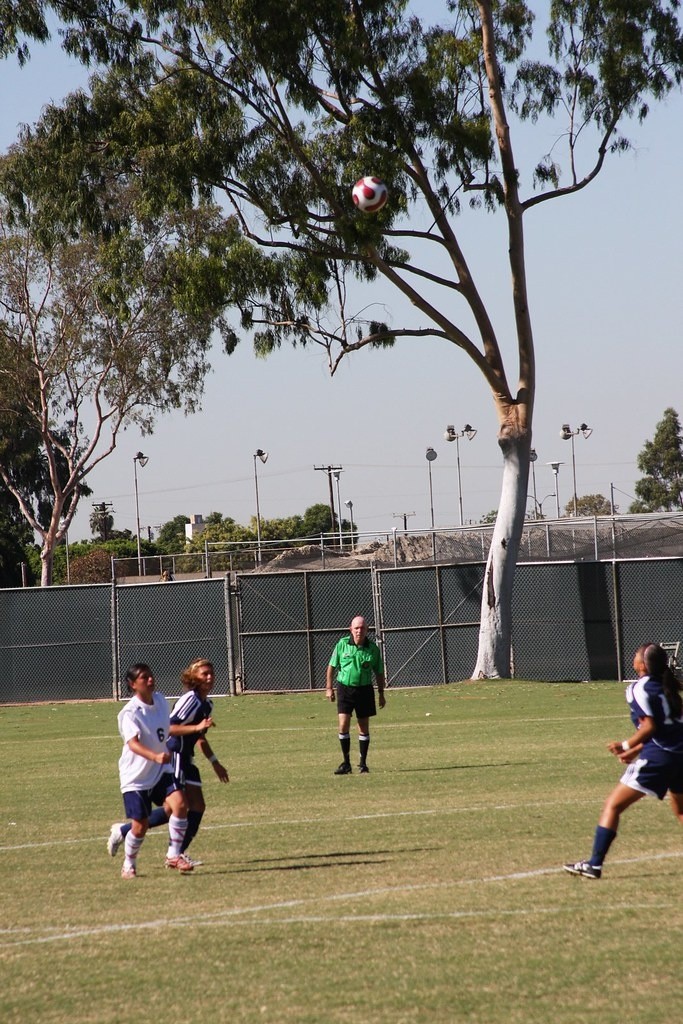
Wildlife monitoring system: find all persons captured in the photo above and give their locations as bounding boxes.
[326,616,386,774]
[108,659,229,867]
[117,664,194,878]
[560,646,683,880]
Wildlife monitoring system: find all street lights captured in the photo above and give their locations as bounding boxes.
[344,500,355,551]
[527,493,557,519]
[132,451,150,576]
[425,446,438,528]
[443,423,478,534]
[545,461,566,518]
[328,468,347,550]
[529,448,538,520]
[252,448,269,566]
[560,422,594,517]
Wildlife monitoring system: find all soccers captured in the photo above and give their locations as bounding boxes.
[352,176,388,214]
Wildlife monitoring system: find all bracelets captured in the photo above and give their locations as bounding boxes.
[622,741,629,751]
[209,755,216,762]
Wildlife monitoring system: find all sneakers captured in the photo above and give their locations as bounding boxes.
[106,823,125,857]
[563,860,602,879]
[335,763,352,774]
[164,854,194,870]
[181,853,202,865]
[120,865,136,879]
[357,765,369,773]
